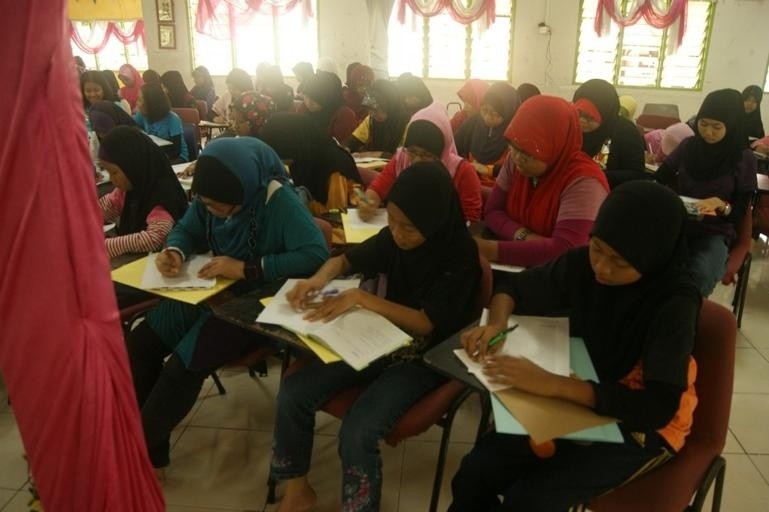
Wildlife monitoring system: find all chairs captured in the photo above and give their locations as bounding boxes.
[85,99,768,511]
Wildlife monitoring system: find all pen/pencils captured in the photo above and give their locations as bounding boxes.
[302,289,338,302]
[472,324,518,356]
[160,233,177,277]
[353,187,378,216]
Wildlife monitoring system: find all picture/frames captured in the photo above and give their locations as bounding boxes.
[154,1,174,22]
[157,22,178,49]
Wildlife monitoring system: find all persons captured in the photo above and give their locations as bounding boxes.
[269,158,487,511]
[125,136,329,490]
[445,179,705,511]
[73,57,768,309]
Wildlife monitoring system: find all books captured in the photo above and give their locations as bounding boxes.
[254,285,416,372]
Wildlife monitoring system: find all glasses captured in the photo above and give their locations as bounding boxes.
[402,147,437,161]
[576,109,596,122]
[189,192,235,215]
